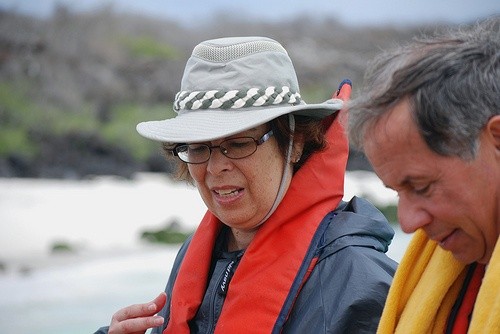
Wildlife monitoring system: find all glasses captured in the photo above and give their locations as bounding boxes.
[172,130,275,163]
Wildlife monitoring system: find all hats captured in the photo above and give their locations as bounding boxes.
[136,35,343,144]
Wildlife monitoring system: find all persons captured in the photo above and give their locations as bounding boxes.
[91,36,399,334]
[336,13,500,334]
